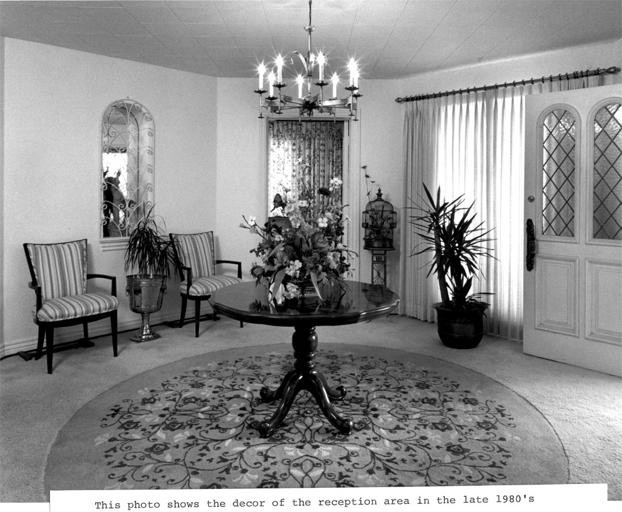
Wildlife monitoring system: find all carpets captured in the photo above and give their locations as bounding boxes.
[43,341,571,503]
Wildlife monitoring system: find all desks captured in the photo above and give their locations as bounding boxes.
[208,282,400,437]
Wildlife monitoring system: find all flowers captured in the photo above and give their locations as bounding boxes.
[239,177,359,314]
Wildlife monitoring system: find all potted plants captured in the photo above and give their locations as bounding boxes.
[123,203,170,342]
[401,181,501,348]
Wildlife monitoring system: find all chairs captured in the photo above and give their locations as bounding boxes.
[169,231,243,337]
[23,238,119,374]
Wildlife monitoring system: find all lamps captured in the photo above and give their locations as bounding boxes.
[254,0,364,121]
